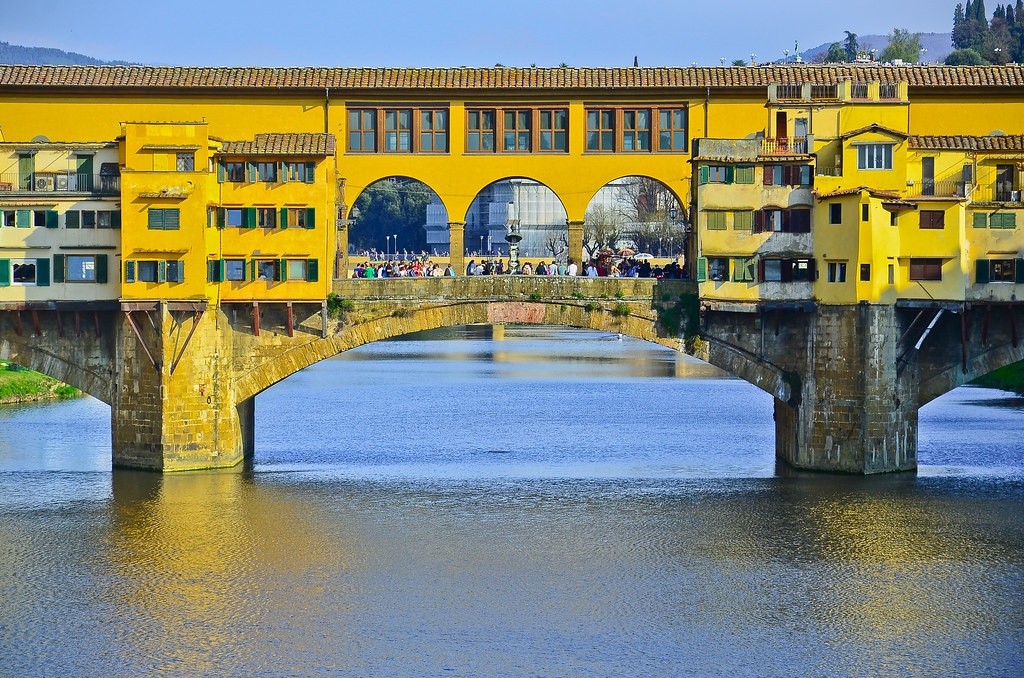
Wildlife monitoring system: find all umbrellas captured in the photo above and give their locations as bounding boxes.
[634,253,654,260]
[617,249,635,255]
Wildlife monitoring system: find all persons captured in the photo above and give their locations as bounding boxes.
[466,259,577,276]
[584,258,688,278]
[354,247,455,278]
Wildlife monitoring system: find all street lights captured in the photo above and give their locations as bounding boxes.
[670,237,673,257]
[720,56,727,65]
[659,237,662,257]
[994,48,1001,65]
[394,234,397,253]
[505,224,523,276]
[870,49,878,62]
[480,235,483,256]
[783,49,789,64]
[386,236,390,261]
[922,49,927,65]
